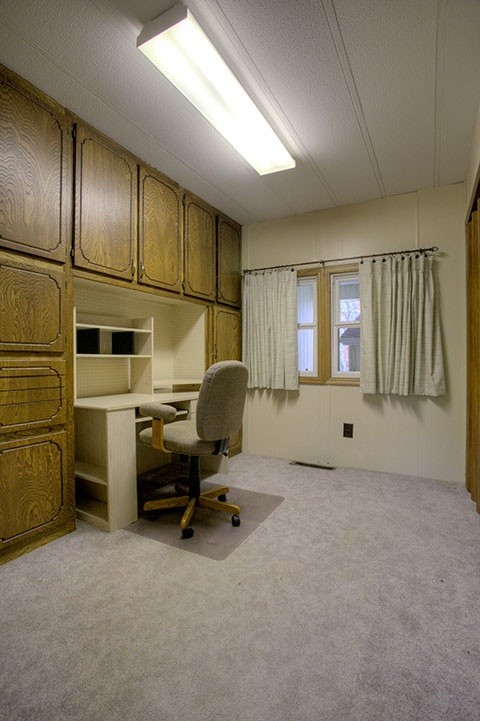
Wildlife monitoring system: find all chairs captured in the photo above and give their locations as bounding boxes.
[139,360,249,538]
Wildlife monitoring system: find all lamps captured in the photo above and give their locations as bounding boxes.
[135,5,296,177]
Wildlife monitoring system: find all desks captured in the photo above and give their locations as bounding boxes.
[73,391,228,532]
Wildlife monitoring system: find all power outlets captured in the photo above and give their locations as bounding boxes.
[343,423,353,438]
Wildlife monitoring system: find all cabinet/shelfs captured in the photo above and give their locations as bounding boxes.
[206,305,243,459]
[1,64,241,308]
[0,257,76,566]
[73,308,154,400]
